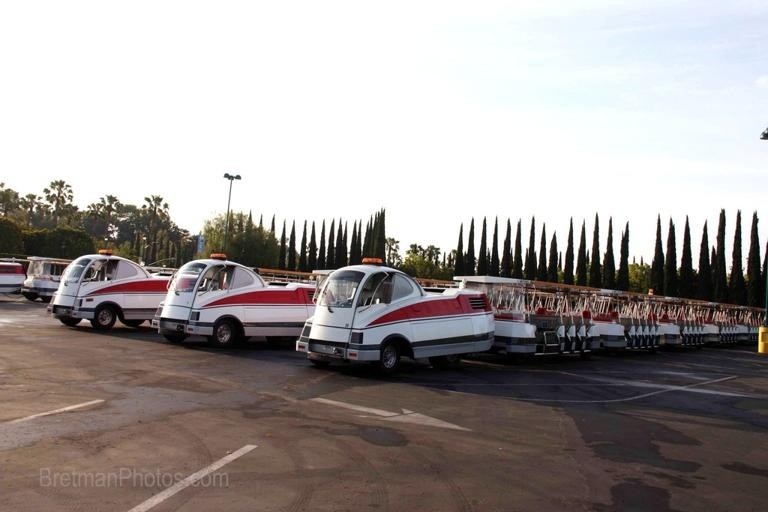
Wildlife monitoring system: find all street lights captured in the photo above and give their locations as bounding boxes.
[224,173,242,249]
[142,235,147,259]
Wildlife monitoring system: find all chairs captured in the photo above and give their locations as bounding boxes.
[361,283,385,305]
